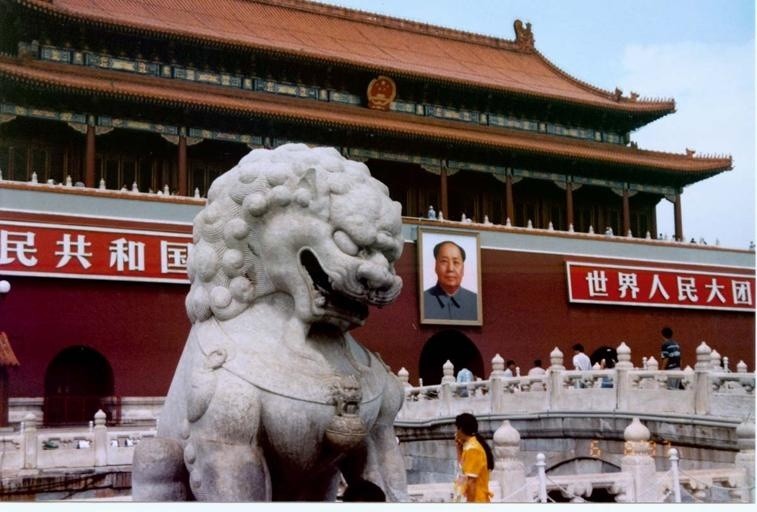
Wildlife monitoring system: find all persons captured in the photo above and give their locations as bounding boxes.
[525,359,547,392]
[454,362,475,395]
[571,343,594,388]
[424,241,478,320]
[454,412,492,503]
[660,326,684,388]
[600,353,617,388]
[502,359,517,392]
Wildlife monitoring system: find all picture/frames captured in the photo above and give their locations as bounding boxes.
[416,225,484,328]
